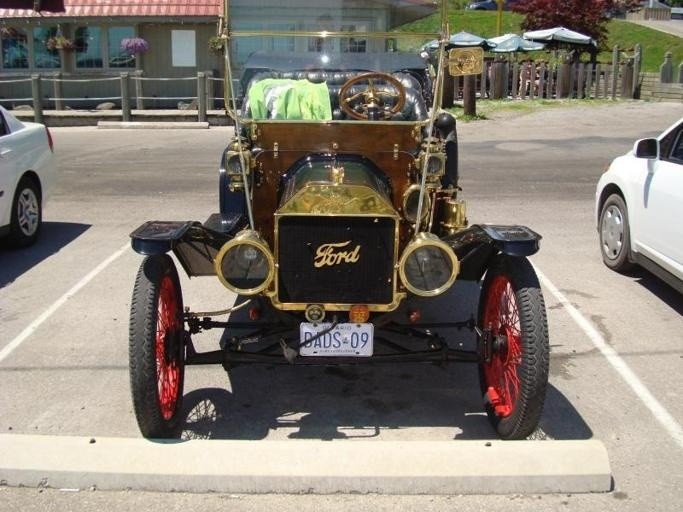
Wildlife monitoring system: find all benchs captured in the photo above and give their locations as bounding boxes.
[236,71,429,137]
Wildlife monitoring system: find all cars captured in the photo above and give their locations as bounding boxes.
[0,104,55,249]
[3,48,128,71]
[592,117,683,298]
[126,12,553,443]
[469,0,514,12]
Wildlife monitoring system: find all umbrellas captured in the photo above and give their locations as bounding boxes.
[421,39,439,51]
[487,33,544,61]
[522,25,599,67]
[437,28,495,50]
